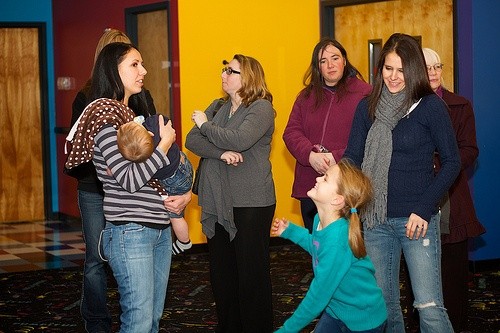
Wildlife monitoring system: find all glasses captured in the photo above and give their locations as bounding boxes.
[426,63,444,73]
[222,67,241,75]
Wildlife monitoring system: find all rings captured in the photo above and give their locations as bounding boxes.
[418,226,423,228]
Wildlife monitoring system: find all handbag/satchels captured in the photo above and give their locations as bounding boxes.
[192,98,225,195]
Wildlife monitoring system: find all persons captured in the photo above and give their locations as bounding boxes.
[407,47,483,333]
[282,39,375,233]
[63,29,156,333]
[272,158,388,333]
[185,54,277,333]
[90,42,193,333]
[117,114,193,256]
[336,32,459,333]
